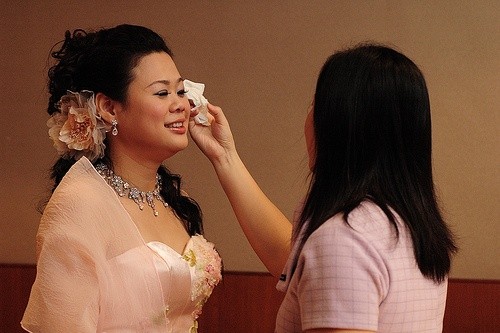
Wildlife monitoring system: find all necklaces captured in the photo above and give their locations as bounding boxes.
[95,162,168,216]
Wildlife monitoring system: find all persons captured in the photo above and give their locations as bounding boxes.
[19,23,225,333]
[187,37,463,333]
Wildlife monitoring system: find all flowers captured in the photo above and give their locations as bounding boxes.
[46,89,106,163]
